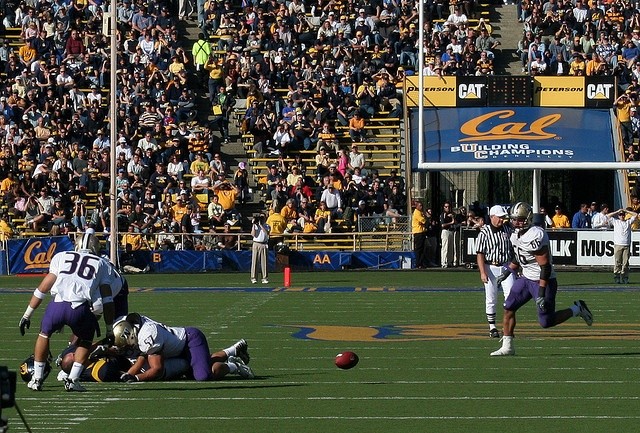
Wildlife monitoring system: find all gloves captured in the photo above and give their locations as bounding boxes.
[18,313,31,336]
[119,370,139,383]
[536,297,546,311]
[105,325,115,348]
[496,273,507,289]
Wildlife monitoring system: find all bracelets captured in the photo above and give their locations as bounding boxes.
[165,187,170,190]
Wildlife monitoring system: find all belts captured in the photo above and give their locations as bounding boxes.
[253,241,267,244]
[484,261,503,266]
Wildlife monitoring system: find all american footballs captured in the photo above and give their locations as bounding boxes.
[335,351,359,369]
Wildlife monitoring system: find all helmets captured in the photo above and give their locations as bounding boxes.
[509,202,534,230]
[74,233,102,256]
[20,350,52,384]
[108,320,138,356]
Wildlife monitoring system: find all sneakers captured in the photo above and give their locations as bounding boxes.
[574,299,594,326]
[489,328,499,338]
[63,378,87,392]
[251,279,258,284]
[489,347,515,356]
[623,278,630,283]
[228,356,255,379]
[233,338,250,365]
[27,378,44,391]
[262,280,269,284]
[614,278,620,283]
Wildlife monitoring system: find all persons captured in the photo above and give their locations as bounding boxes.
[628,105,640,133]
[136,101,160,128]
[266,165,282,192]
[50,105,65,128]
[54,128,72,151]
[1,13,15,28]
[88,36,109,57]
[292,155,306,180]
[91,191,111,232]
[190,170,209,193]
[201,0,220,36]
[35,61,49,84]
[43,87,56,105]
[108,313,250,382]
[99,57,111,85]
[588,201,600,216]
[18,135,36,151]
[20,171,34,195]
[250,213,271,284]
[569,52,586,76]
[596,31,609,45]
[490,202,593,356]
[189,151,209,176]
[39,187,54,215]
[96,151,111,177]
[516,1,573,34]
[175,122,191,138]
[25,90,39,107]
[137,131,159,154]
[57,159,74,182]
[536,29,545,43]
[520,31,534,72]
[622,40,640,68]
[79,53,99,80]
[455,206,468,226]
[171,196,191,222]
[630,194,640,209]
[116,136,134,158]
[289,111,312,150]
[280,198,298,221]
[11,76,25,97]
[19,38,37,66]
[118,1,133,26]
[47,55,60,69]
[266,206,288,236]
[133,204,147,223]
[623,144,640,162]
[538,207,552,228]
[549,54,569,76]
[528,34,547,60]
[591,204,613,231]
[571,204,591,228]
[579,32,596,54]
[597,36,619,67]
[35,117,52,140]
[142,190,159,218]
[69,84,86,110]
[336,148,350,176]
[220,1,500,114]
[4,84,12,97]
[141,33,154,55]
[612,94,635,152]
[529,54,549,74]
[1,170,21,195]
[8,89,22,104]
[20,7,40,33]
[558,29,576,51]
[82,158,100,179]
[269,182,289,211]
[32,30,54,55]
[72,149,88,175]
[73,257,129,346]
[132,6,153,38]
[20,233,115,391]
[426,208,439,268]
[118,130,137,144]
[127,154,144,184]
[60,352,255,383]
[212,180,239,213]
[169,43,190,63]
[156,32,169,49]
[0,115,9,133]
[53,151,73,171]
[467,211,484,228]
[170,31,182,48]
[17,149,36,171]
[24,104,43,121]
[349,111,365,143]
[188,130,208,153]
[68,113,85,142]
[66,182,85,200]
[24,188,44,230]
[159,126,175,140]
[40,145,58,161]
[620,0,639,39]
[15,1,27,25]
[606,209,638,283]
[61,54,80,76]
[54,5,70,33]
[19,114,34,131]
[165,137,187,158]
[118,54,200,129]
[626,76,640,93]
[43,16,56,38]
[5,126,19,143]
[11,98,27,120]
[192,33,212,92]
[46,171,61,192]
[343,167,407,223]
[476,205,519,337]
[5,136,19,154]
[210,154,228,179]
[157,224,175,248]
[156,5,176,37]
[6,61,20,82]
[412,202,428,268]
[86,85,103,107]
[211,85,237,145]
[586,53,602,76]
[0,96,13,118]
[348,145,366,174]
[550,205,571,228]
[124,31,140,54]
[208,195,225,222]
[71,0,112,30]
[167,154,184,182]
[121,225,142,251]
[0,212,13,243]
[2,147,16,164]
[605,2,625,29]
[291,177,313,201]
[56,69,74,85]
[143,149,162,162]
[52,198,66,222]
[315,147,331,182]
[320,184,344,215]
[92,129,110,151]
[624,206,640,230]
[440,202,455,269]
[150,162,173,194]
[66,30,84,55]
[317,122,341,153]
[274,154,289,179]
[549,36,569,64]
[272,125,289,151]
[88,109,103,131]
[182,205,193,243]
[0,39,15,64]
[315,202,331,234]
[631,61,640,84]
[286,165,302,187]
[69,200,87,229]
[588,1,604,28]
[573,0,588,24]
[297,198,312,227]
[5,182,26,214]
[234,162,249,206]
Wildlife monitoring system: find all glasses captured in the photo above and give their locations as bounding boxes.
[331,169,335,170]
[555,209,560,210]
[592,204,596,206]
[29,95,33,96]
[258,13,263,14]
[253,103,258,105]
[445,206,450,208]
[619,214,624,216]
[541,211,545,212]
[61,131,65,133]
[104,154,108,155]
[324,126,329,127]
[46,147,51,148]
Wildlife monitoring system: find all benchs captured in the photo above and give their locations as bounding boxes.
[235,111,401,177]
[6,28,26,54]
[78,89,111,106]
[1,193,247,252]
[284,220,410,250]
[624,142,640,190]
[432,3,493,35]
[273,89,289,100]
[210,35,226,54]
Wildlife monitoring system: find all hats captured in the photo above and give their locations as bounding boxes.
[340,77,346,81]
[217,173,225,175]
[180,190,187,195]
[0,96,6,101]
[193,125,202,133]
[327,164,338,169]
[185,204,194,208]
[359,200,366,205]
[96,129,105,135]
[124,189,130,194]
[555,205,561,208]
[8,136,13,140]
[22,114,28,120]
[29,188,38,193]
[60,68,66,72]
[291,165,298,170]
[118,137,126,143]
[343,56,349,60]
[13,89,18,93]
[352,144,358,148]
[340,16,346,20]
[40,61,46,65]
[250,31,256,34]
[358,18,365,21]
[359,8,366,13]
[37,13,43,18]
[9,125,16,128]
[328,184,334,187]
[446,45,453,50]
[617,210,625,214]
[179,122,186,126]
[22,150,28,155]
[297,111,303,116]
[490,205,508,217]
[353,110,359,114]
[356,31,362,36]
[45,144,51,147]
[4,39,9,43]
[338,30,344,33]
[433,65,441,72]
[173,137,180,142]
[171,30,178,34]
[302,198,308,202]
[143,102,152,106]
[380,68,387,73]
[398,66,404,71]
[278,47,284,52]
[316,80,322,86]
[298,83,303,87]
[409,24,416,28]
[91,84,97,88]
[276,17,283,21]
[320,146,325,150]
[118,169,126,172]
[271,165,276,168]
[306,47,318,54]
[337,149,346,154]
[9,54,15,57]
[161,6,168,12]
[239,162,246,169]
[228,55,237,60]
[15,76,22,80]
[176,196,182,200]
[55,197,61,202]
[329,12,335,16]
[287,98,292,103]
